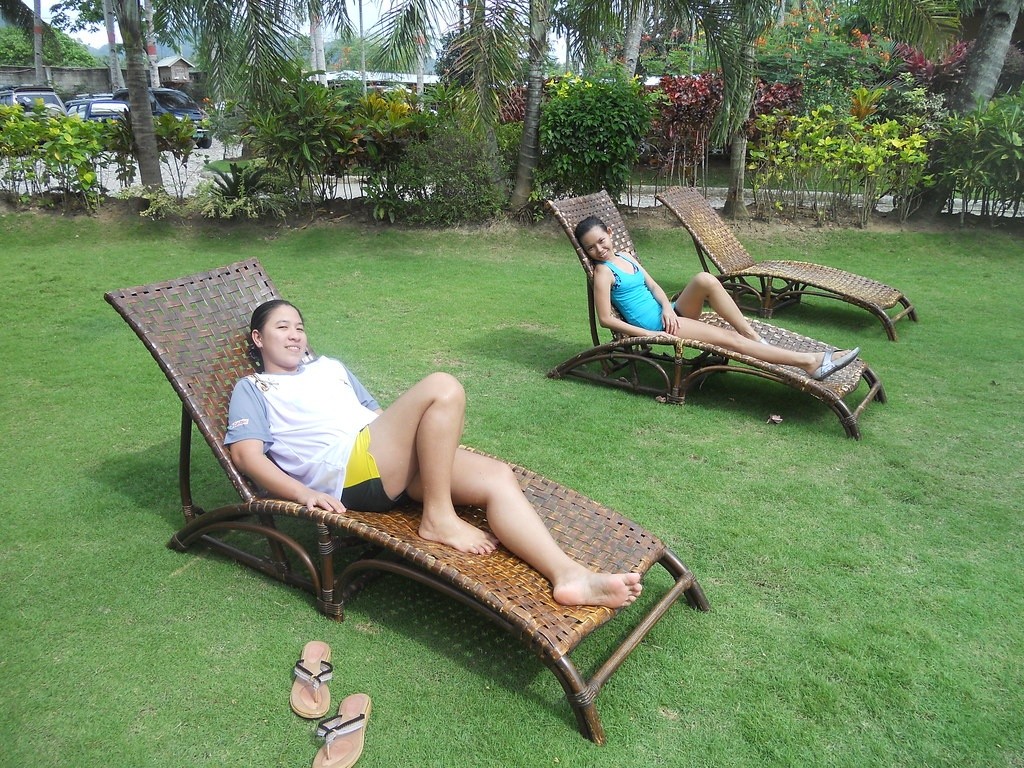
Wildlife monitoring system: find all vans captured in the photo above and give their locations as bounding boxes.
[112,87,213,151]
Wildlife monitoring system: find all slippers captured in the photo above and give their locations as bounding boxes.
[312,693,371,768]
[814,347,860,380]
[290,641,333,719]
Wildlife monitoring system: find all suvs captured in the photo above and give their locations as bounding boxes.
[0,83,69,121]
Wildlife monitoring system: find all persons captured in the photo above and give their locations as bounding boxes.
[576,215,860,381]
[225,300,642,610]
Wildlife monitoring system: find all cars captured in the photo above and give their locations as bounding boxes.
[65,100,129,124]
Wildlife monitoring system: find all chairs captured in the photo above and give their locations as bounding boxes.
[654,185,921,343]
[104,255,711,746]
[544,190,887,442]
